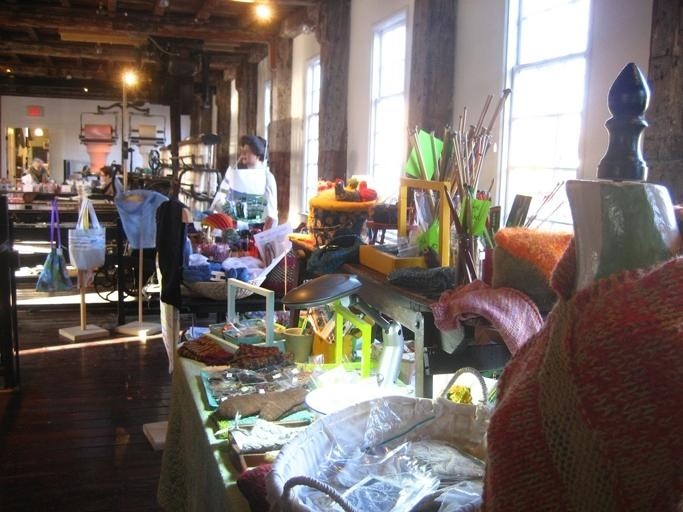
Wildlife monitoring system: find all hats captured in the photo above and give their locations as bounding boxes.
[238,134,267,162]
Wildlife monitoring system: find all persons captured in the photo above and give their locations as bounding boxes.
[28,157,50,185]
[209,134,278,231]
[99,165,117,199]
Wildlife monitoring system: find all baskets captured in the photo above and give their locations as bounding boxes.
[182,274,266,299]
[272,393,494,512]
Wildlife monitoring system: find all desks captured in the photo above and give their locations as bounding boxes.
[175,295,290,325]
[286,231,491,402]
[155,341,497,512]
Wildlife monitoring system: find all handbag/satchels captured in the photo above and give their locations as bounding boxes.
[35,254,72,293]
[68,229,105,270]
